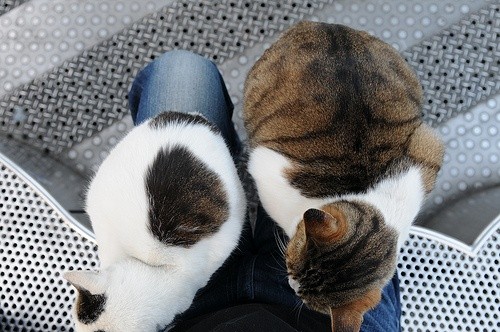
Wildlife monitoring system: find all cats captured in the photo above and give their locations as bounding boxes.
[62,112,246,332]
[245,21,445,332]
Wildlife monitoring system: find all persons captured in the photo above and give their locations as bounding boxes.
[128,50,401,332]
